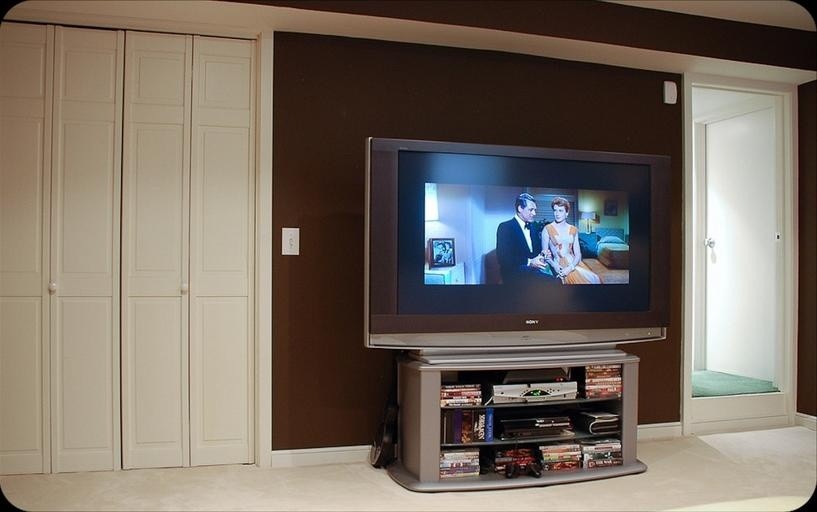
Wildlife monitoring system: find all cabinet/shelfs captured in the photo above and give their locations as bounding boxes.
[0,22,256,477]
[385,353,648,494]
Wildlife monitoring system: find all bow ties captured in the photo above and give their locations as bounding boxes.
[525,221,531,230]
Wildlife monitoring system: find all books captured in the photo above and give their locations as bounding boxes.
[437,362,623,478]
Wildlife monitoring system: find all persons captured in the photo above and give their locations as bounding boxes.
[541,196,603,286]
[433,242,446,263]
[495,192,561,286]
[441,241,454,265]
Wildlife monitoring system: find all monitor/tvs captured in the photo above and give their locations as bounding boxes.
[364,136,671,365]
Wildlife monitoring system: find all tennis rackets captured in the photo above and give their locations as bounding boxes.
[370,403,397,467]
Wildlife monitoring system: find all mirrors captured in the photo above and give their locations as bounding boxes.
[679,71,800,436]
